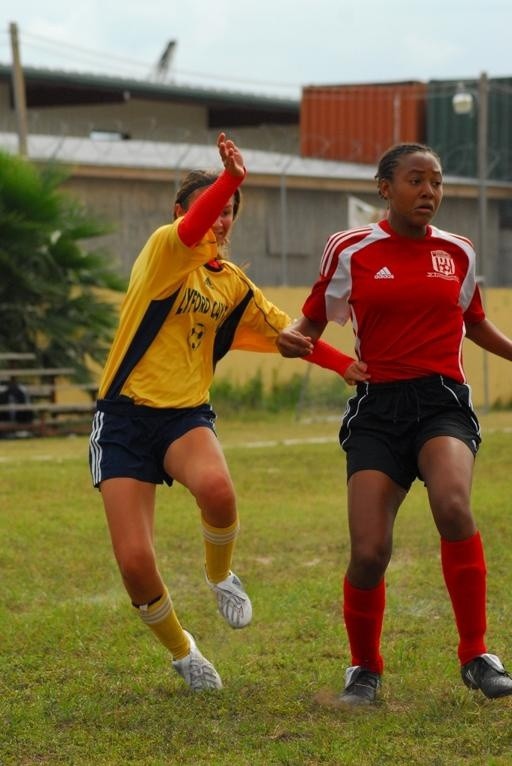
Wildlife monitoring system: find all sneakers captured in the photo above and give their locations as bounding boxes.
[204,559,253,629]
[340,665,383,709]
[458,652,512,699]
[171,629,222,697]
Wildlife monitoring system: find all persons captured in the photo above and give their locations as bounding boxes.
[87,132,374,694]
[278,141,510,702]
